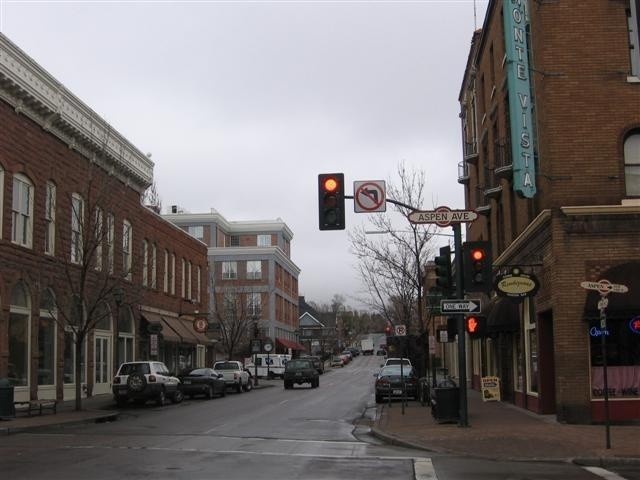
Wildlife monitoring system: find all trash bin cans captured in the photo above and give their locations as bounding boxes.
[0,378,16,418]
[431,387,461,423]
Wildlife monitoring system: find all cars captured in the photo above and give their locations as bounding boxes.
[181,367,228,399]
[330,346,361,368]
[212,360,253,393]
[373,358,421,404]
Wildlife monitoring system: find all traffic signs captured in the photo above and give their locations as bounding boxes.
[438,299,482,315]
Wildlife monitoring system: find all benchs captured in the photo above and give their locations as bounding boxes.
[13,398,58,419]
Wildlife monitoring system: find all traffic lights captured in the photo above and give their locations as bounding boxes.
[434,245,454,296]
[462,240,493,292]
[467,317,486,340]
[317,172,346,231]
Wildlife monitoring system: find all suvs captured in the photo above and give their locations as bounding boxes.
[283,359,320,390]
[111,359,184,405]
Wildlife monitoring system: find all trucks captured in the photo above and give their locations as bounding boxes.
[243,353,293,380]
